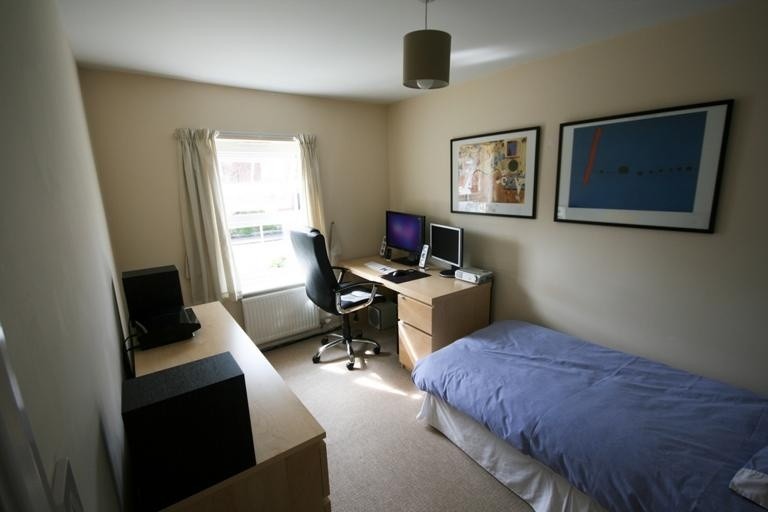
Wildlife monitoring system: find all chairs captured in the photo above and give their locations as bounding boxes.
[289,224,386,371]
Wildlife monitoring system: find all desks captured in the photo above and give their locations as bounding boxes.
[340,256,494,370]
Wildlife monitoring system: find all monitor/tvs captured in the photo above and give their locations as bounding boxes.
[429,221,464,278]
[386,210,425,266]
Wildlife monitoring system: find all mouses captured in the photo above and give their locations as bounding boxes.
[392,269,406,277]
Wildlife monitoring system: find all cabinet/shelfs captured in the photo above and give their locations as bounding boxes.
[128,300,333,512]
[396,282,492,370]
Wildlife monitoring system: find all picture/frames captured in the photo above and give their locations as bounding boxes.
[450,99,735,234]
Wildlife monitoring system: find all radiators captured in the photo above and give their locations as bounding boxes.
[240,285,331,346]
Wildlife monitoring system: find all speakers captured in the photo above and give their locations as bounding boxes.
[379,235,388,256]
[418,244,430,268]
[120,350,257,512]
[367,302,397,330]
[121,264,184,329]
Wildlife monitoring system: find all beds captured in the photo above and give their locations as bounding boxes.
[412,319,768,512]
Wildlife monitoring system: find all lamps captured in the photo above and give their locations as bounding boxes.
[403,1,451,91]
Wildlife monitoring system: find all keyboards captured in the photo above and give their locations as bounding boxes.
[364,260,397,275]
